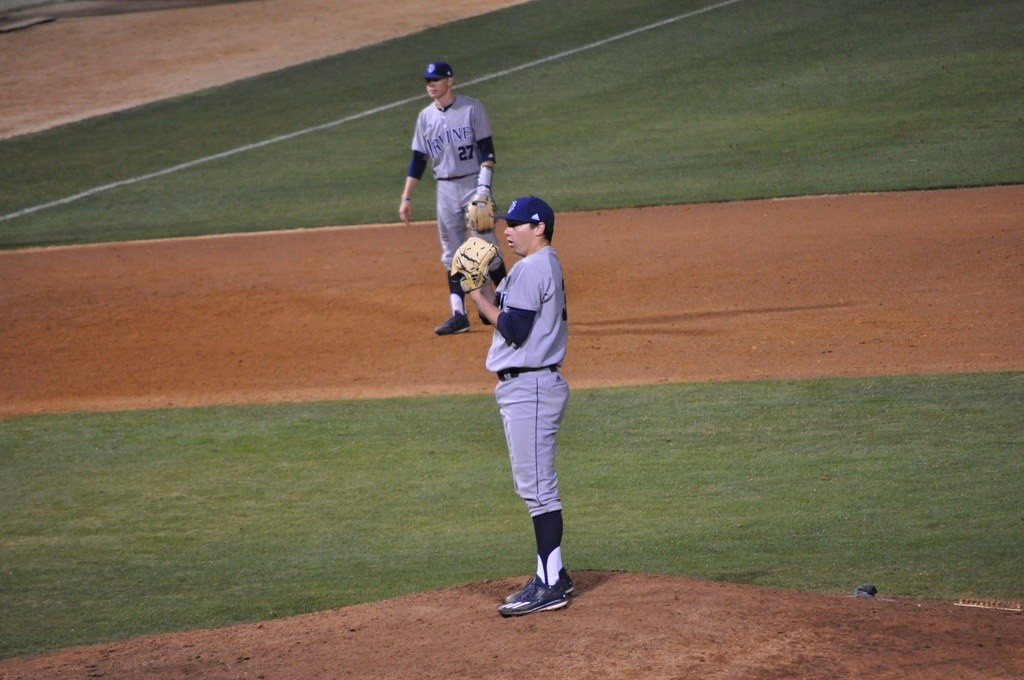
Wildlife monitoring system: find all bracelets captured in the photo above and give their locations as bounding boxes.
[401,198,410,201]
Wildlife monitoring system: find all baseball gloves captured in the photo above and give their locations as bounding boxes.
[449,235,500,294]
[465,191,499,235]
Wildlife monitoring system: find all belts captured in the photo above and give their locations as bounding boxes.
[498,365,556,381]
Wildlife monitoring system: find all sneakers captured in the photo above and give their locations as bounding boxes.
[434,311,470,334]
[499,573,567,614]
[503,567,575,603]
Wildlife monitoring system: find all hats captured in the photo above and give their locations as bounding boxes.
[491,196,555,227]
[421,61,453,79]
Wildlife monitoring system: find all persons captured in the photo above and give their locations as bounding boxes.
[399,61,507,335]
[451,196,575,617]
